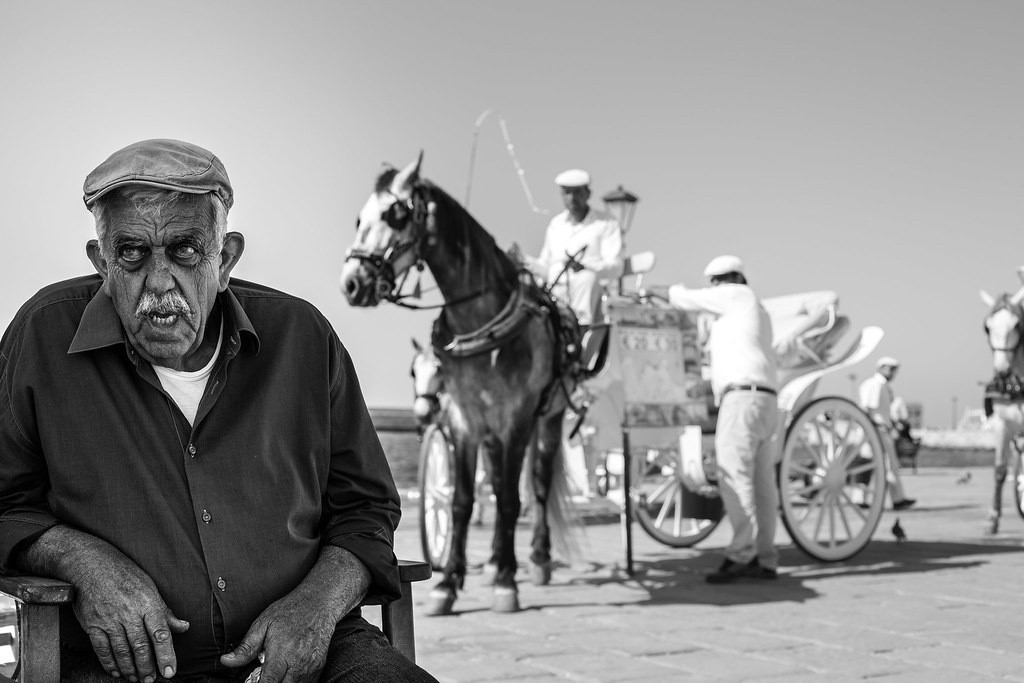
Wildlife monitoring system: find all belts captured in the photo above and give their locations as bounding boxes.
[725,385,777,394]
[992,349,1015,351]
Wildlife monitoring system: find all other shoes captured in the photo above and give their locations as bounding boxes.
[706,558,743,584]
[750,567,777,581]
[894,499,915,509]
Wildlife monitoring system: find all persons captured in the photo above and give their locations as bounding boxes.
[0,139,439,683]
[633,256,782,584]
[857,356,918,511]
[509,169,625,380]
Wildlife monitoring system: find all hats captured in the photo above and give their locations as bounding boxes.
[878,356,899,367]
[82,138,234,215]
[704,255,743,276]
[554,169,590,188]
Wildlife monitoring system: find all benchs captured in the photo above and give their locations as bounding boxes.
[763,287,841,367]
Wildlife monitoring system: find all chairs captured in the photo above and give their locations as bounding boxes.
[605,251,655,307]
[0,558,434,683]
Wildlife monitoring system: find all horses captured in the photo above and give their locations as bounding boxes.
[338,148,583,617]
[976,283,1023,536]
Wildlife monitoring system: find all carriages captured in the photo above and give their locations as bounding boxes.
[335,148,896,625]
[406,335,633,574]
[976,263,1024,538]
